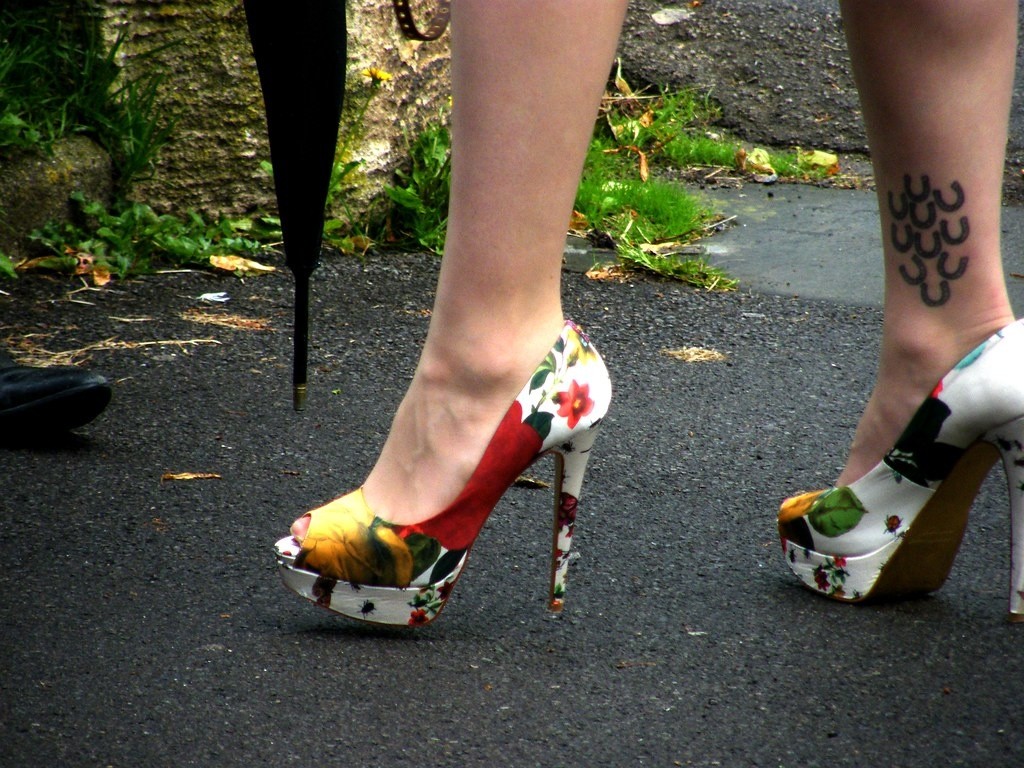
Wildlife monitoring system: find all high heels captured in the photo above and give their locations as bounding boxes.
[778,318,1024,622]
[272,317,613,625]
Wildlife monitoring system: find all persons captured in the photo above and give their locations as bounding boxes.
[0,346,112,435]
[272,0,1023,621]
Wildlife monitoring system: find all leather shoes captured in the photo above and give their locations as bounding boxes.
[0,347,112,438]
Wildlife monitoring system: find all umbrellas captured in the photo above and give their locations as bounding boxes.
[243,0,347,411]
